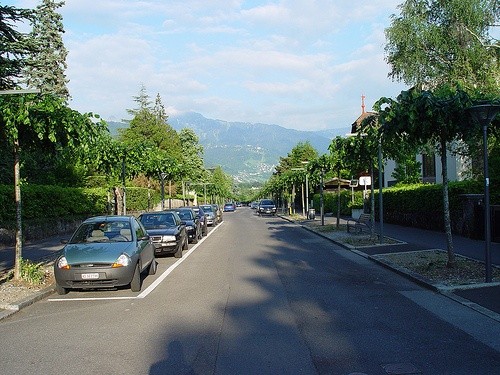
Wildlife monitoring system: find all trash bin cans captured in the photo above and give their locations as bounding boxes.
[309,209,315,220]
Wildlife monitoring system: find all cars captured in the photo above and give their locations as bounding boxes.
[54,215,157,295]
[248,203,252,207]
[179,206,208,236]
[199,204,218,227]
[163,208,202,244]
[224,203,235,212]
[236,204,241,207]
[210,204,223,223]
[136,211,189,258]
[251,202,258,209]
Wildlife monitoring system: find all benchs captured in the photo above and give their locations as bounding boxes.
[347,213,373,235]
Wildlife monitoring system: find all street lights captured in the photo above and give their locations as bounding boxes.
[465,103,500,284]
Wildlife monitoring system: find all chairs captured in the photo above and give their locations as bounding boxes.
[86,230,109,243]
[116,229,133,242]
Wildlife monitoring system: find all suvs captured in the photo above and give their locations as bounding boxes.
[256,199,277,216]
